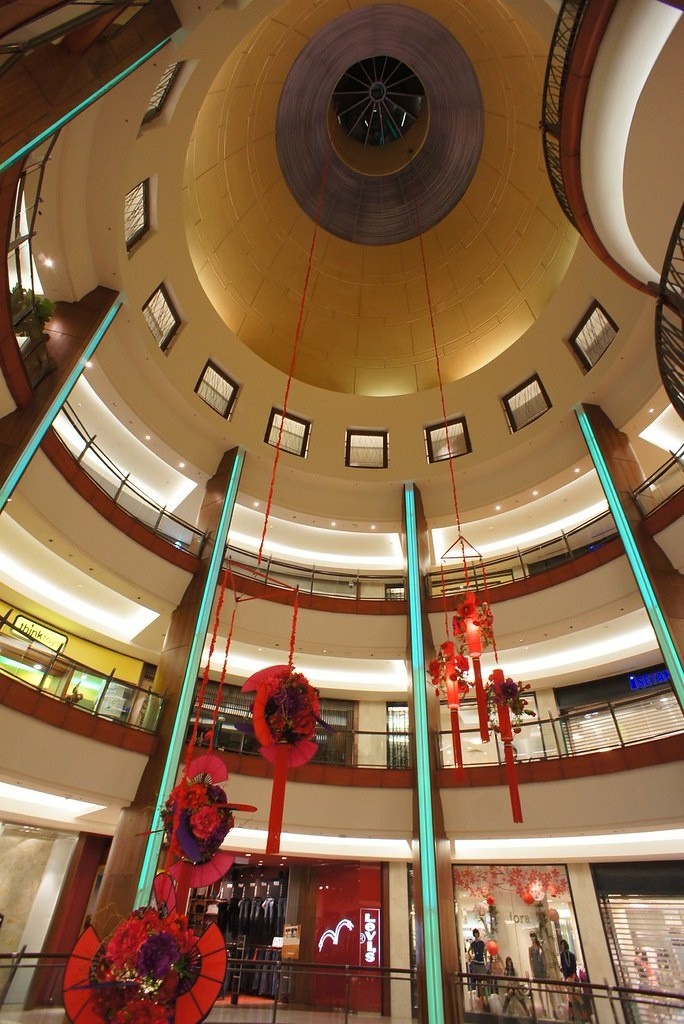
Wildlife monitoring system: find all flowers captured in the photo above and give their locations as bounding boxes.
[92,905,200,1024]
[458,599,478,619]
[160,777,235,864]
[472,601,494,648]
[458,679,478,703]
[484,674,536,734]
[234,677,337,744]
[432,676,446,697]
[426,656,446,676]
[453,643,470,676]
[449,673,458,681]
[452,616,467,637]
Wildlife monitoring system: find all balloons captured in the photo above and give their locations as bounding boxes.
[522,893,533,905]
[549,907,558,922]
[487,941,498,957]
[487,896,494,907]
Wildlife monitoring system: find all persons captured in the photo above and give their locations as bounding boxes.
[559,939,578,1022]
[467,928,488,998]
[501,956,531,1018]
[528,931,559,1020]
